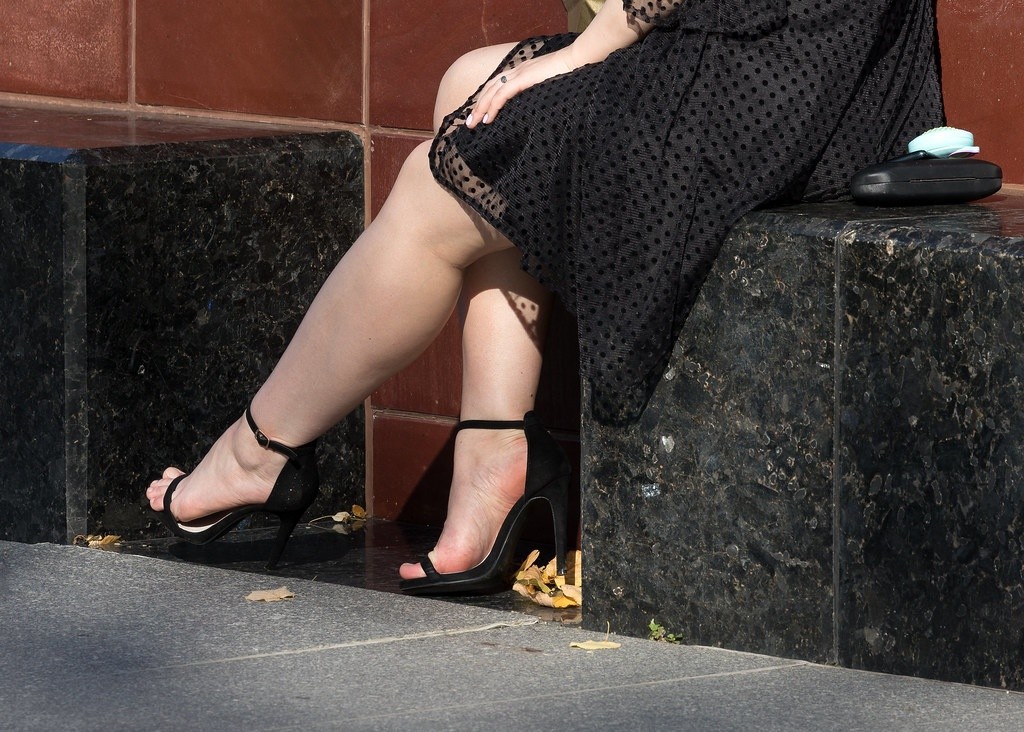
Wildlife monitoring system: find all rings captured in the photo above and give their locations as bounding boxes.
[501,76,507,83]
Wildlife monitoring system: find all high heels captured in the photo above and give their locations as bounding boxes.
[395,413,567,600]
[161,404,319,558]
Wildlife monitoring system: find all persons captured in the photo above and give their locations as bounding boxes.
[146,0,947,592]
